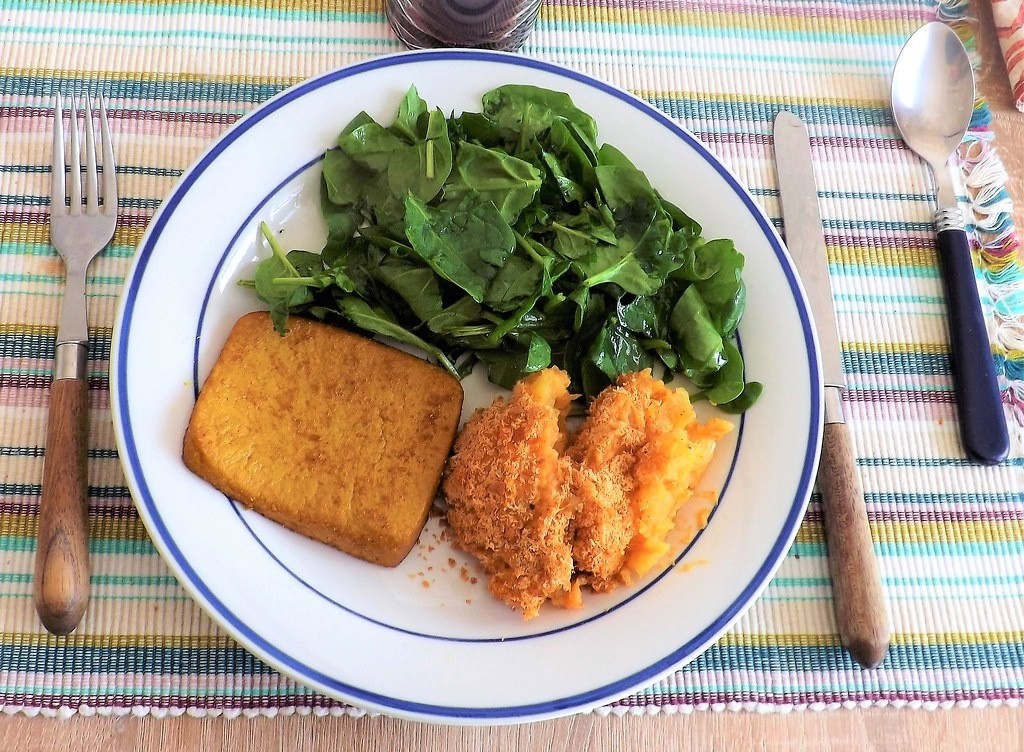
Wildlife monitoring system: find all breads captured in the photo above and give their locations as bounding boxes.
[182,312,463,567]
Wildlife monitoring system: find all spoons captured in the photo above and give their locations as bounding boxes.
[890,22,1010,466]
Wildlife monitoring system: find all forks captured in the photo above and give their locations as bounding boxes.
[36,93,118,634]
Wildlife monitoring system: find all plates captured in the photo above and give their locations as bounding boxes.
[109,48,826,727]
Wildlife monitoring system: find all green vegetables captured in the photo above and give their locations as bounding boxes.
[239,83,763,415]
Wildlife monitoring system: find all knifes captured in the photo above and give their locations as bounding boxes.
[773,110,889,669]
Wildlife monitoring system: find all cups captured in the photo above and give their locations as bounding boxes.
[384,0,543,52]
